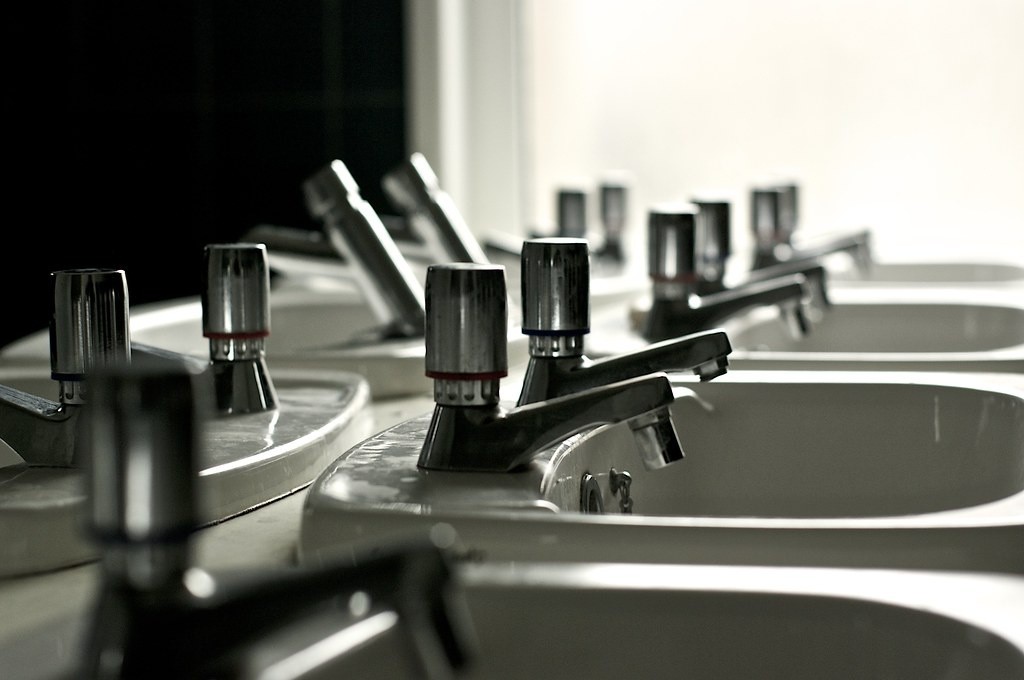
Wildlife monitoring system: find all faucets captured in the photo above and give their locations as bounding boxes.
[417,263,683,474]
[364,149,517,312]
[57,357,486,680]
[745,183,875,274]
[247,158,427,343]
[0,266,136,474]
[127,240,283,421]
[645,197,832,345]
[512,236,733,408]
[478,178,631,280]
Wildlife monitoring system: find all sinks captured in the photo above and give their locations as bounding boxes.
[538,381,1024,519]
[129,301,387,357]
[258,579,1024,680]
[729,303,1024,353]
[0,373,65,471]
[815,261,1024,288]
